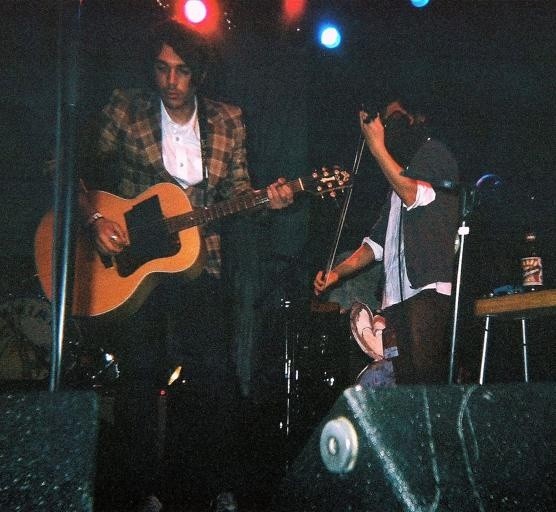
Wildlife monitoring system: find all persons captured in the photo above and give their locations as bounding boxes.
[312,92,461,386]
[43,18,294,511]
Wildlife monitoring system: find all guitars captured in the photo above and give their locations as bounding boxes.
[35,164,351,323]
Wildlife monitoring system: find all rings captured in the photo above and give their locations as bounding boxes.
[282,195,288,203]
[109,235,118,242]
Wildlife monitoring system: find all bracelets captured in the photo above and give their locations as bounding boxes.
[84,213,104,225]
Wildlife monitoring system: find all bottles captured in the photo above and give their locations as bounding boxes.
[521,232,544,293]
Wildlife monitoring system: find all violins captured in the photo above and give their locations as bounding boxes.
[360,111,425,171]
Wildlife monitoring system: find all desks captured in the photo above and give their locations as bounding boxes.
[473,288,556,385]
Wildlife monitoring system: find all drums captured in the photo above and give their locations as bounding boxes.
[1,290,90,387]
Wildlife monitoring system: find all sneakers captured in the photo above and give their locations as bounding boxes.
[209,491,240,512]
[134,494,163,512]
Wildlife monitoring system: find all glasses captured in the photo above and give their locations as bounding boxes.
[381,110,409,121]
